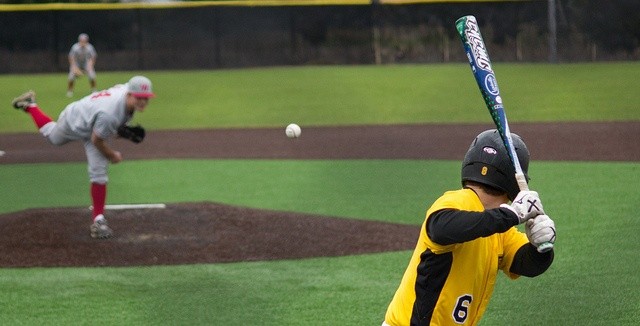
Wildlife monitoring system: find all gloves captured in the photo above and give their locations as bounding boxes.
[525,215,556,247]
[500,190,544,224]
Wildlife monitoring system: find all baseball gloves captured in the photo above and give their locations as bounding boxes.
[117,125,145,143]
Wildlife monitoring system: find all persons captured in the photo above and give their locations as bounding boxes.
[14,76,157,238]
[67,33,97,97]
[381,129,556,326]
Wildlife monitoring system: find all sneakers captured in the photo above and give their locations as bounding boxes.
[12,90,35,109]
[90,218,113,238]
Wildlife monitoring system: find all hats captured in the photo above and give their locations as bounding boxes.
[127,76,157,98]
[78,33,89,42]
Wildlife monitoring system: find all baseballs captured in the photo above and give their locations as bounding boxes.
[285,124,301,138]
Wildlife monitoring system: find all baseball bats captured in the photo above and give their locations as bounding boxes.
[454,15,553,254]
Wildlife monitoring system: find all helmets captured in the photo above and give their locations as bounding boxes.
[462,128,529,202]
